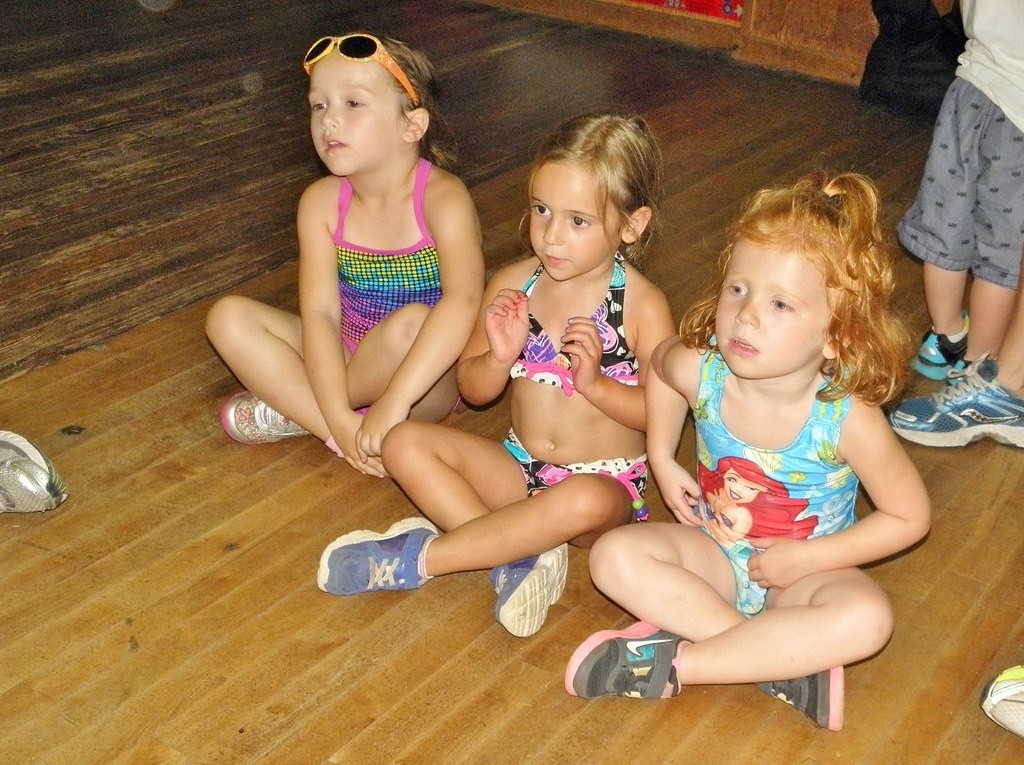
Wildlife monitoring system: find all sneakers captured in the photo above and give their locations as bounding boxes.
[489,543,568,637]
[888,350,1024,447]
[317,517,438,596]
[220,391,312,445]
[0,430,69,513]
[565,621,695,699]
[757,666,844,730]
[980,665,1024,739]
[911,311,973,386]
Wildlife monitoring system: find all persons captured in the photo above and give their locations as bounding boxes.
[880,0,1024,394]
[560,164,934,734]
[311,109,676,639]
[198,30,483,482]
[885,234,1024,455]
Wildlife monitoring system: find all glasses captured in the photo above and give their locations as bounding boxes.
[304,34,419,106]
